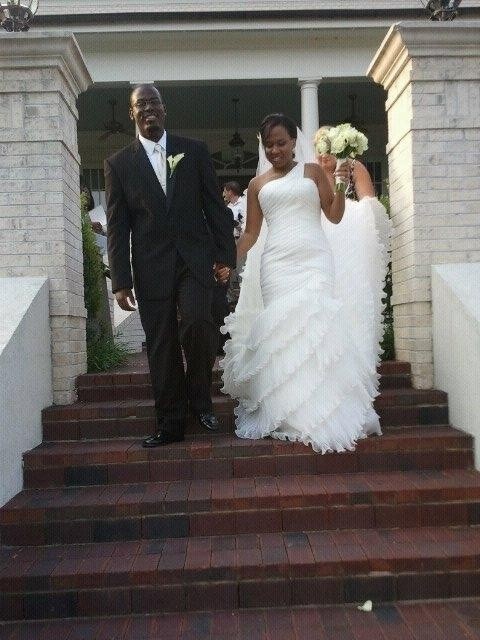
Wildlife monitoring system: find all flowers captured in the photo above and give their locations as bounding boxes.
[313,122,369,193]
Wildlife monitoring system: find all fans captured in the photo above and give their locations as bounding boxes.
[97,98,135,140]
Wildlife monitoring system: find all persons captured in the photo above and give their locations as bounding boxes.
[80,184,113,280]
[221,180,251,304]
[213,111,396,453]
[313,125,375,203]
[102,81,236,451]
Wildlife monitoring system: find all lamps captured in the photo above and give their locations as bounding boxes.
[0,0,39,32]
[228,97,245,172]
[421,0,462,21]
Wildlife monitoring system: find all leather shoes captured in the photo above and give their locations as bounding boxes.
[198,410,219,431]
[143,430,184,446]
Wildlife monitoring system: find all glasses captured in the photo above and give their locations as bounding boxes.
[132,99,162,108]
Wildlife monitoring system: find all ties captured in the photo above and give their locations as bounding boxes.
[154,145,167,196]
